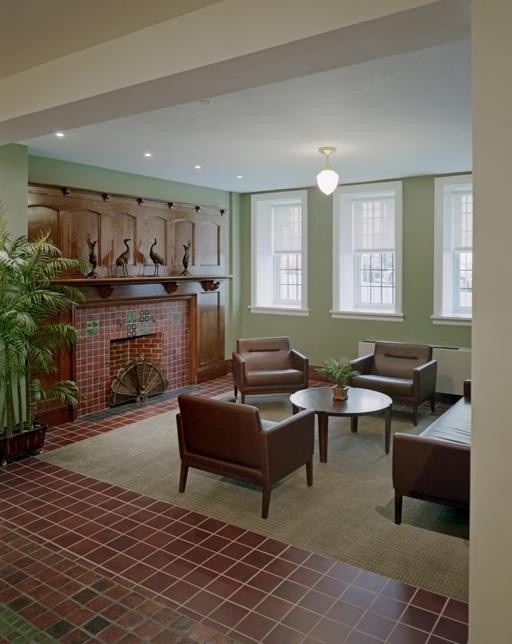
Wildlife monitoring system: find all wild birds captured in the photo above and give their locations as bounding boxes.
[148,237,168,277]
[116,238,133,278]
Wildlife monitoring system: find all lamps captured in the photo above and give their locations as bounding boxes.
[316,146,341,195]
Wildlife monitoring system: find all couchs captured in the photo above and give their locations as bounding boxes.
[394,380,469,523]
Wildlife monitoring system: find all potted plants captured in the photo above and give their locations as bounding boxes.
[0,205,83,464]
[317,357,358,401]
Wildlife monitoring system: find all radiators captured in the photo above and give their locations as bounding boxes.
[358,339,471,397]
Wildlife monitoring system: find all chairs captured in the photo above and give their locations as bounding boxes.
[231,337,310,403]
[174,394,317,519]
[347,343,440,426]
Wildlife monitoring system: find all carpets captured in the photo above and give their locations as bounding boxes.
[36,391,471,603]
